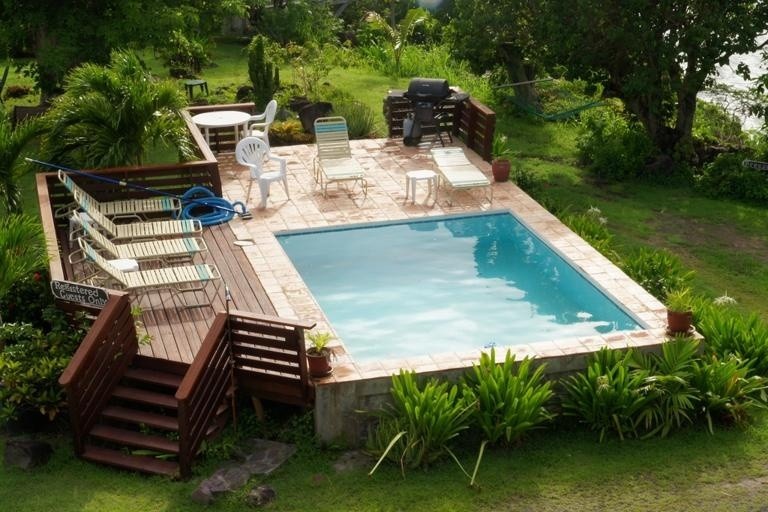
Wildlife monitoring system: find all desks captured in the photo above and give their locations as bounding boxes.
[186,80,208,99]
[406,170,439,202]
[193,111,252,146]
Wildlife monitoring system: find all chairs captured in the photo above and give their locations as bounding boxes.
[239,99,278,150]
[431,147,494,207]
[313,116,368,198]
[236,137,292,208]
[54,169,223,310]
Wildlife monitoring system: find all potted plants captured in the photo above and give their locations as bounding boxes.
[663,286,696,337]
[305,331,338,377]
[490,133,517,182]
